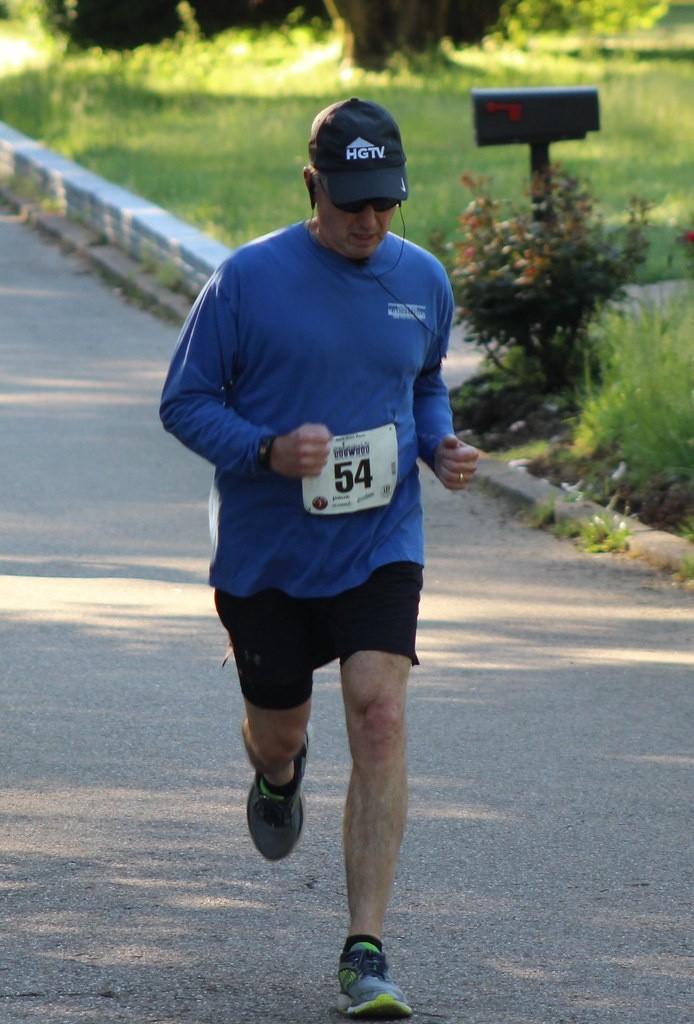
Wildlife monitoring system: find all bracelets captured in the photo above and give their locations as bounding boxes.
[258,434,275,469]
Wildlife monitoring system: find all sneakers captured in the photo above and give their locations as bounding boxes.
[327,949,411,1017]
[245,732,310,862]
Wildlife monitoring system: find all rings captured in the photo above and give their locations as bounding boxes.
[459,473,463,482]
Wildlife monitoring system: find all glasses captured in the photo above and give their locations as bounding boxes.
[315,172,403,214]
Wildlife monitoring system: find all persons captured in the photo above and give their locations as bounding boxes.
[160,97,479,1020]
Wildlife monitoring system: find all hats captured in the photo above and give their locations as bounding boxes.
[308,97,409,205]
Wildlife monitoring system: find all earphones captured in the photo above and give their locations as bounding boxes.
[308,175,316,210]
